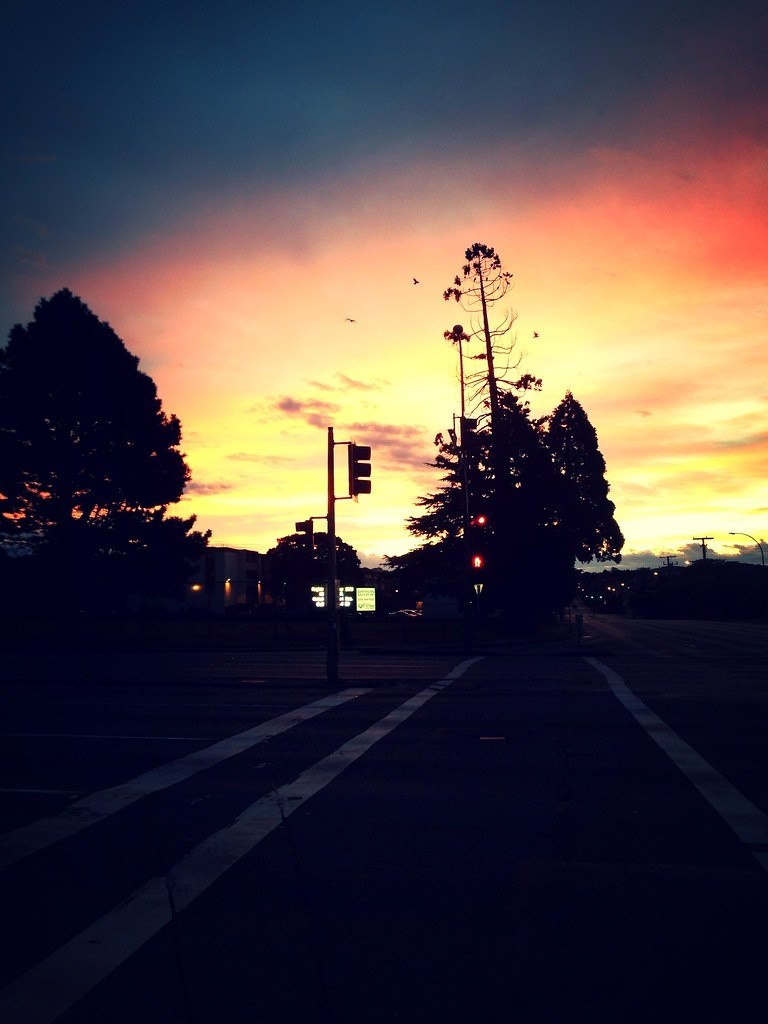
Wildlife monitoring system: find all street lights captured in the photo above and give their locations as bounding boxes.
[730,532,765,565]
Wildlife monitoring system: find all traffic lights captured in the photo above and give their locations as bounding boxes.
[296,521,314,562]
[471,552,485,571]
[348,443,372,496]
[475,515,489,527]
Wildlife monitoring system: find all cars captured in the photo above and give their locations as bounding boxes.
[388,608,423,619]
